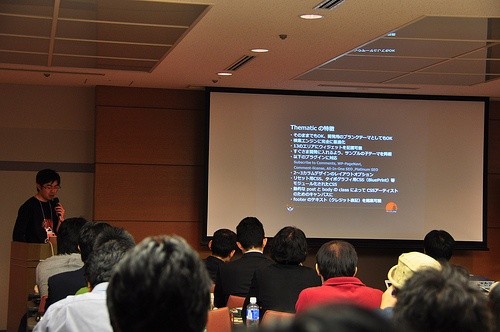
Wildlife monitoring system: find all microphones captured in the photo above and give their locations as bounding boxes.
[54,197,61,220]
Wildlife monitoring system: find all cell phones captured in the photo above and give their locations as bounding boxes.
[384,279,396,295]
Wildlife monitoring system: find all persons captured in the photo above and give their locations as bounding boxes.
[12,169,65,243]
[31,216,499,332]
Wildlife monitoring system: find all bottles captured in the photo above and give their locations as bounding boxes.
[246,297,259,332]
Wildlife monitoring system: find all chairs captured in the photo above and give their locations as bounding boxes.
[19,292,295,332]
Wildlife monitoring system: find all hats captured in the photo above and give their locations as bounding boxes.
[388,252,442,289]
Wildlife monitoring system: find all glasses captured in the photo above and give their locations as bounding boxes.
[43,185,61,189]
